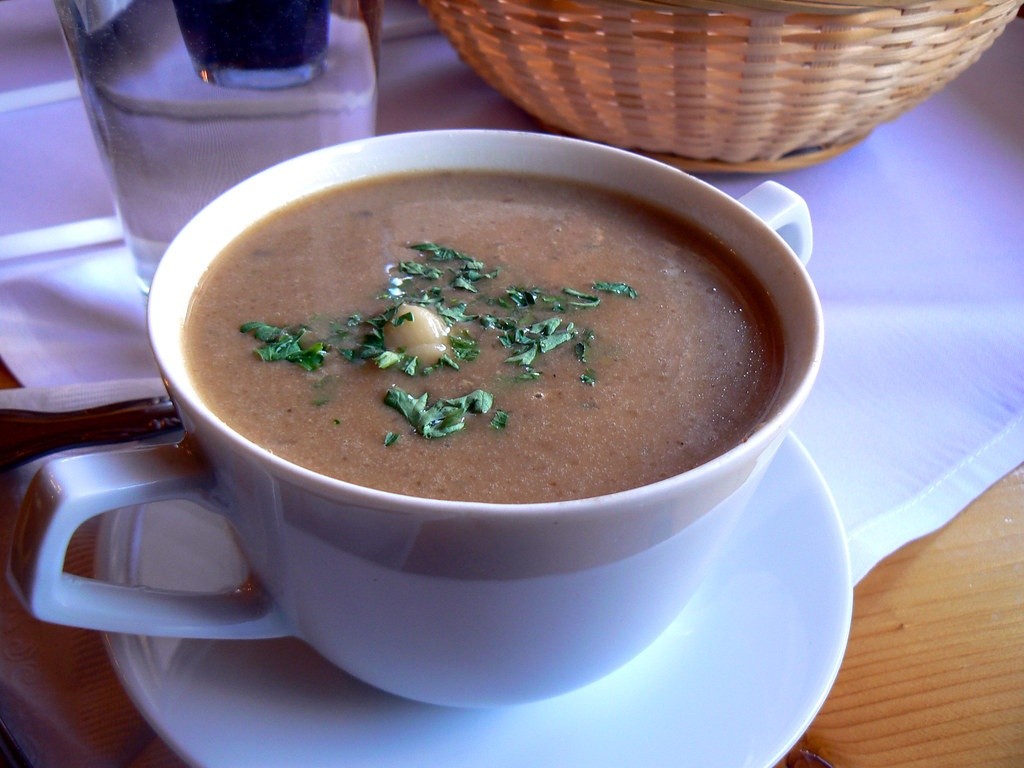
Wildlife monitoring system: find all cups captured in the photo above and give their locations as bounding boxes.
[55,0,379,301]
[6,126,829,714]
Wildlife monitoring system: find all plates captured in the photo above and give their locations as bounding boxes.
[90,427,857,768]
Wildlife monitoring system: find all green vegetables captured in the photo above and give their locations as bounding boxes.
[240,240,640,448]
